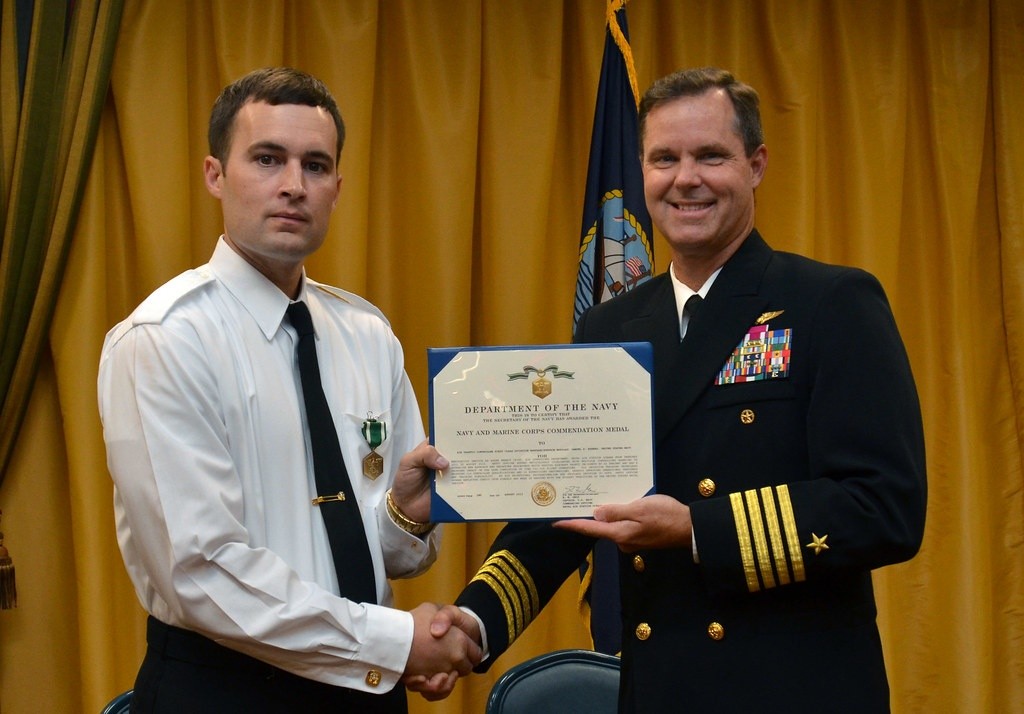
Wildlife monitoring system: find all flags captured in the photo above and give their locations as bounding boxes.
[571,0,654,656]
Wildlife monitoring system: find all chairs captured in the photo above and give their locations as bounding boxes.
[484,649,620,714]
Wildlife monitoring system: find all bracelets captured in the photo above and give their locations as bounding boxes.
[387,494,434,534]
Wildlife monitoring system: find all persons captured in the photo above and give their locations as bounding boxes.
[95,66,480,714]
[407,65,928,714]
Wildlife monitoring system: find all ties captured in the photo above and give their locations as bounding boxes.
[285,300,377,604]
[680,294,705,352]
